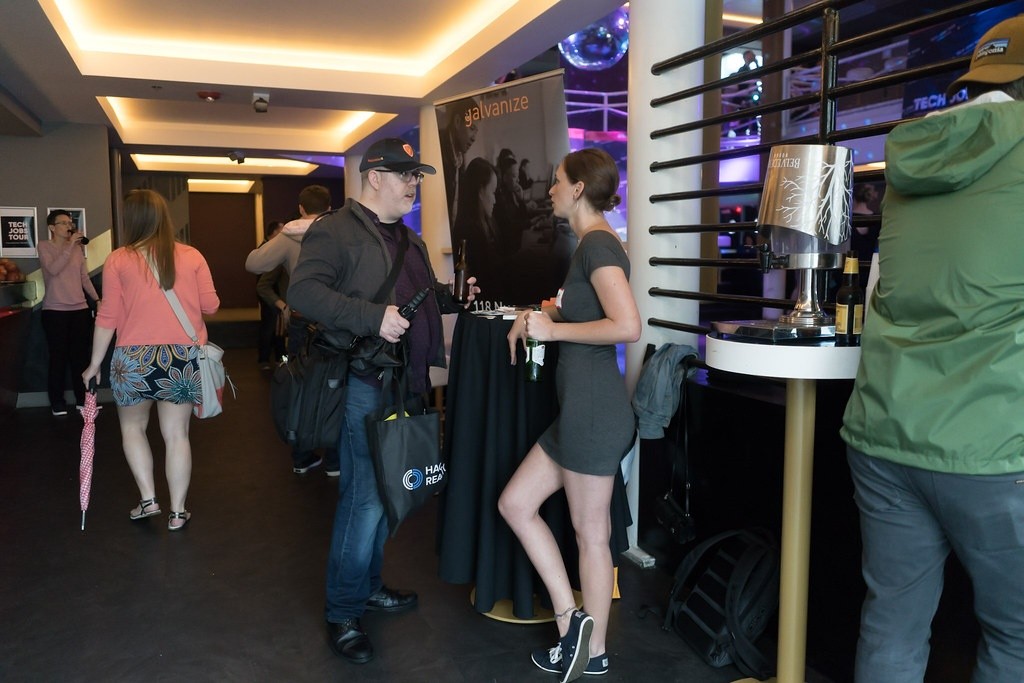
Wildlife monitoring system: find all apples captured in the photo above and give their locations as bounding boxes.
[0,258,21,280]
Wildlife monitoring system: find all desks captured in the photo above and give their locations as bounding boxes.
[436,309,634,625]
[704,334,862,683]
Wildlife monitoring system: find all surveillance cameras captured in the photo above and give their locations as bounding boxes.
[226,150,246,164]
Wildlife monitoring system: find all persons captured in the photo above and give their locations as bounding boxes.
[735,51,761,136]
[842,12,1024,683]
[497,147,642,683]
[852,180,885,290]
[245,186,342,476]
[37,209,103,415]
[81,189,220,531]
[290,138,449,664]
[439,98,554,308]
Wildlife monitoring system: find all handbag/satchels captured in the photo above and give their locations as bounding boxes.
[267,340,348,448]
[371,384,440,523]
[195,341,238,420]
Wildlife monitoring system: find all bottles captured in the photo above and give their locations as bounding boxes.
[451,240,469,303]
[524,306,546,382]
[67,229,89,245]
[835,249,864,347]
[866,248,880,316]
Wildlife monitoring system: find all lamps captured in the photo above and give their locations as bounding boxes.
[254,98,269,113]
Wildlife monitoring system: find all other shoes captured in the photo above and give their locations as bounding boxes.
[50,402,67,416]
[263,364,271,370]
[76,400,103,410]
[293,452,322,474]
[324,456,341,477]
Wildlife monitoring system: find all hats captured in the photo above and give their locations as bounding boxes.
[359,137,437,175]
[947,14,1023,99]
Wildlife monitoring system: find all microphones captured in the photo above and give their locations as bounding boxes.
[429,288,476,323]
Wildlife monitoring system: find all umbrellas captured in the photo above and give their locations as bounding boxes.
[80,376,99,530]
[349,287,430,378]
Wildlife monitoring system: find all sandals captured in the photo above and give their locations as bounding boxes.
[129,498,162,520]
[167,510,191,531]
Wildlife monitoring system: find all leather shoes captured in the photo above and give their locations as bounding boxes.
[362,585,419,612]
[327,618,374,663]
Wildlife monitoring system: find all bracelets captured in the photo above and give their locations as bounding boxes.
[95,299,101,302]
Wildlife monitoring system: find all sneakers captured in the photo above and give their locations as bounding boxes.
[553,610,594,683]
[531,648,609,674]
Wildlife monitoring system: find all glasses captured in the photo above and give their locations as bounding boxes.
[374,169,424,183]
[52,221,75,227]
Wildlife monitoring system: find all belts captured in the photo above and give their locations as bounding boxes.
[290,310,301,316]
